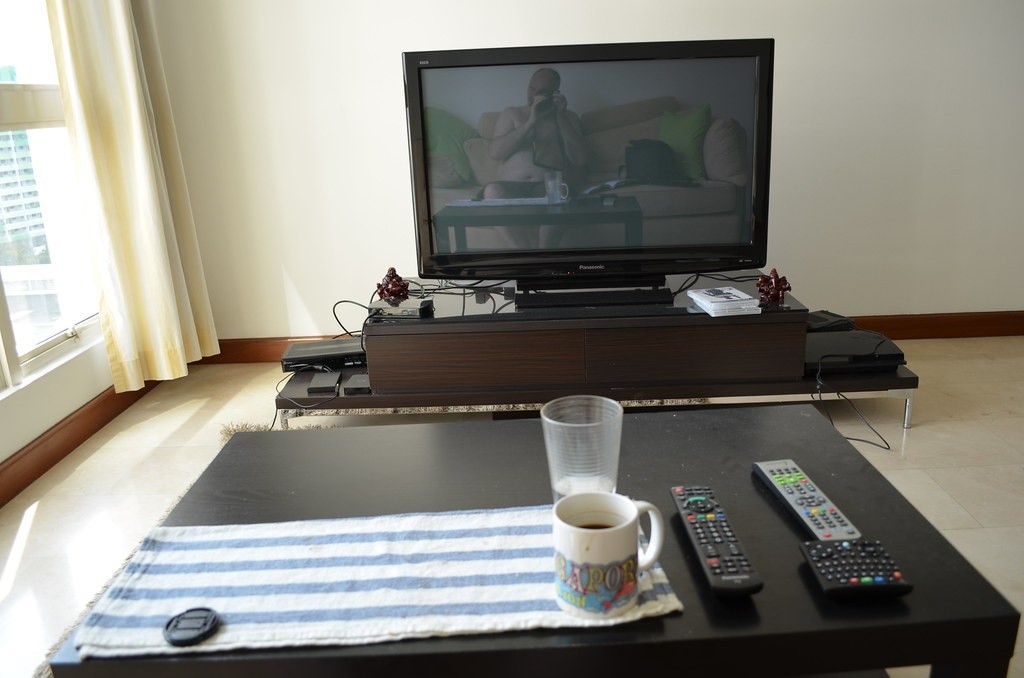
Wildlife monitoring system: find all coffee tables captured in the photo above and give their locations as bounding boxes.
[432,195,643,252]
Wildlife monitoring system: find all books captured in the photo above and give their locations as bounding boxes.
[687,287,762,318]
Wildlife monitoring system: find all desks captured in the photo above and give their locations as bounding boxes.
[50,402,1021,678]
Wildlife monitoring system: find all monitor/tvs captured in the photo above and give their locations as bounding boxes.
[402,38,774,310]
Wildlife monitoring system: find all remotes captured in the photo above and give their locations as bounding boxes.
[752,459,862,542]
[670,485,764,596]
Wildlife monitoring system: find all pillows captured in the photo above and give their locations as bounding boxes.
[423,105,479,180]
[659,103,712,181]
[427,156,462,188]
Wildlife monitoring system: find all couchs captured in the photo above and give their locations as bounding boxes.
[429,97,749,253]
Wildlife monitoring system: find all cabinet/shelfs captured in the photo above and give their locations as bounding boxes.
[276,268,920,428]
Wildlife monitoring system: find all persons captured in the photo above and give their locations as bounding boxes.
[382,267,409,296]
[483,67,589,248]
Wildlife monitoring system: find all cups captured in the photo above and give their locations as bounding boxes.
[540,394,624,506]
[551,492,665,620]
